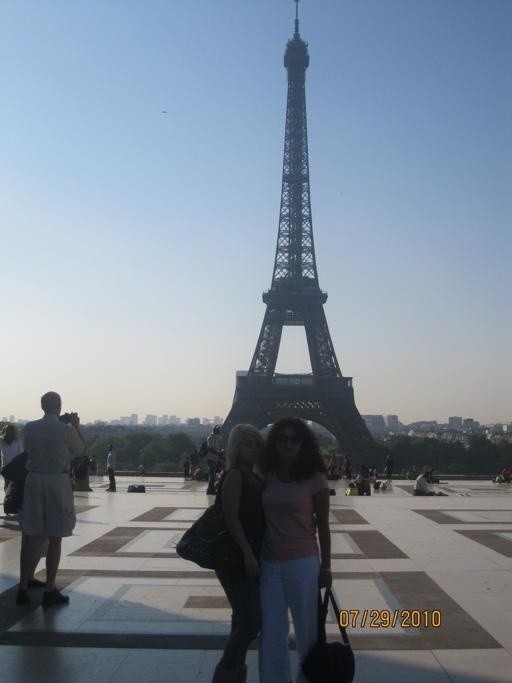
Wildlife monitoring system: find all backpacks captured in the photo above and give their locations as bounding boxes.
[198,442,208,456]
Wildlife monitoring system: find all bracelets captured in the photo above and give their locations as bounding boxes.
[320,566,331,572]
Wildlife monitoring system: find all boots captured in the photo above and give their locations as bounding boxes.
[212,665,247,683]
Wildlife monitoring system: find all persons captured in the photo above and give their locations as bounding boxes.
[16,391,91,607]
[211,424,269,682]
[500,466,511,482]
[414,470,442,496]
[190,448,198,477]
[204,424,223,494]
[1,425,23,519]
[251,416,334,683]
[89,454,96,475]
[323,450,378,496]
[384,455,393,480]
[106,444,116,492]
[183,456,190,476]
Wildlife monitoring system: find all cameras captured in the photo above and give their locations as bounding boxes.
[59,413,71,424]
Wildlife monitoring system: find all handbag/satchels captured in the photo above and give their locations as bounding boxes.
[301,584,355,683]
[175,469,247,574]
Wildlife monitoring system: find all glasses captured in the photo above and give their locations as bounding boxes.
[278,433,300,444]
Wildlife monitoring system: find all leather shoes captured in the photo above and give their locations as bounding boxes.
[16,589,33,604]
[43,588,69,605]
[28,578,46,588]
